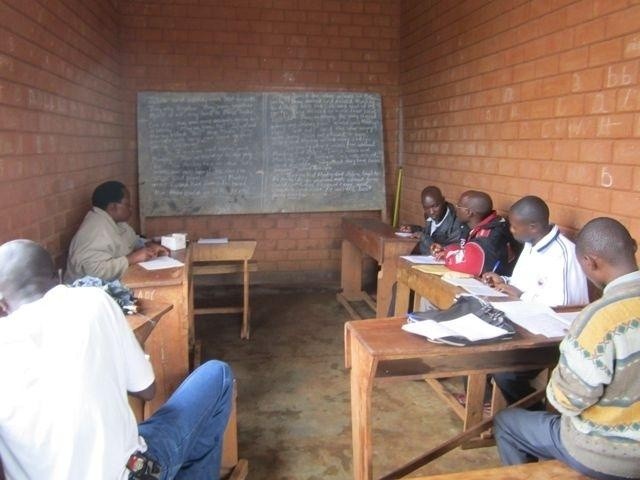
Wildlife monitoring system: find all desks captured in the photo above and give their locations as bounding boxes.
[118,295,174,425]
[179,237,258,338]
[395,254,552,449]
[120,243,201,418]
[338,215,423,321]
[342,304,596,479]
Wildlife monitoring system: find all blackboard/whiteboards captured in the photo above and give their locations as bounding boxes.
[137,89,386,217]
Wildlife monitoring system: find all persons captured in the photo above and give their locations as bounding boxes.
[58,177,175,285]
[424,188,514,281]
[0,235,236,479]
[488,216,640,480]
[478,194,593,308]
[397,185,464,254]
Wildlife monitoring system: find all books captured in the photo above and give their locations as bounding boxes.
[401,264,578,348]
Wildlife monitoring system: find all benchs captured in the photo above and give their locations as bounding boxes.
[418,456,603,478]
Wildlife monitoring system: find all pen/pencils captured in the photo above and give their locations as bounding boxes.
[486,260,501,284]
[138,239,160,260]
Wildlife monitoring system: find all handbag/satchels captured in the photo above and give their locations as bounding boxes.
[406,293,517,347]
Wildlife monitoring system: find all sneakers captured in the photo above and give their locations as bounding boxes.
[452,393,491,408]
